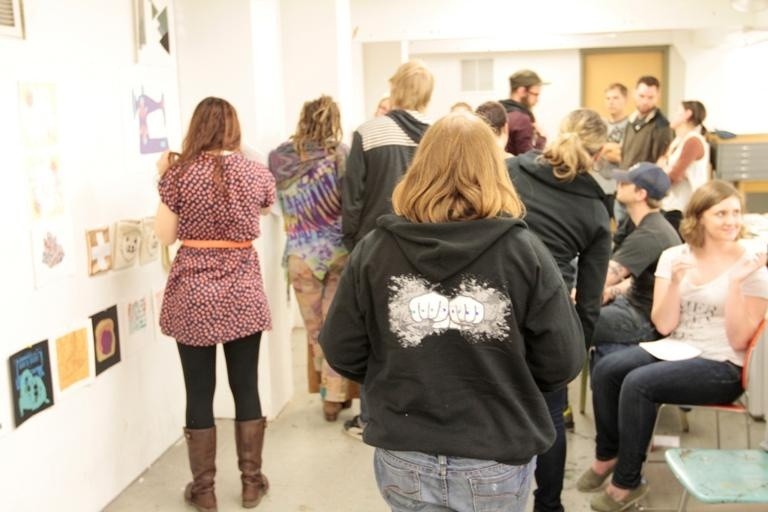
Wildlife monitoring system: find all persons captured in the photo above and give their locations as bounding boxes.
[374,98,389,116]
[268,92,362,422]
[563,162,768,512]
[316,108,584,512]
[339,63,435,437]
[155,96,279,512]
[504,108,618,510]
[474,70,713,250]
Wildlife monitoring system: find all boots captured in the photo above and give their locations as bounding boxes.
[234,416,269,508]
[184,426,217,512]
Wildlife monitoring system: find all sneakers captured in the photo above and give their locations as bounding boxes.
[324,402,369,441]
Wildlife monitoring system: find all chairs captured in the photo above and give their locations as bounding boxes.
[640,310,767,469]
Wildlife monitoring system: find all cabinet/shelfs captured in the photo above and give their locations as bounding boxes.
[707,132,768,217]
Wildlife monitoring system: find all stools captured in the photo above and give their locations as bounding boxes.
[576,343,635,416]
[662,444,767,512]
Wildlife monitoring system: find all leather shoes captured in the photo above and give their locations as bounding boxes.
[577,462,650,512]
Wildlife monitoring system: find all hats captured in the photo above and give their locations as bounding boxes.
[510,70,550,89]
[612,163,671,200]
